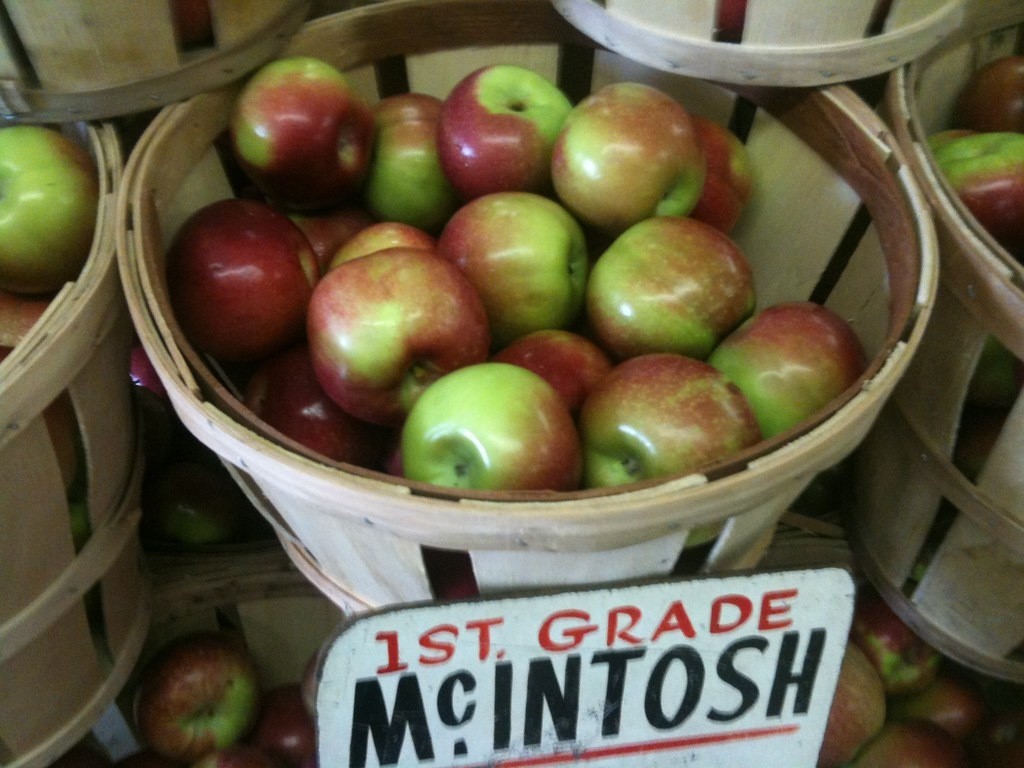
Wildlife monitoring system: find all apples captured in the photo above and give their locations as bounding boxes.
[168,57,865,595]
[1,115,269,552]
[59,630,318,768]
[816,53,1024,768]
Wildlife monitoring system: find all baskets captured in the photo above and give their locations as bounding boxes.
[0,0,1024,768]
